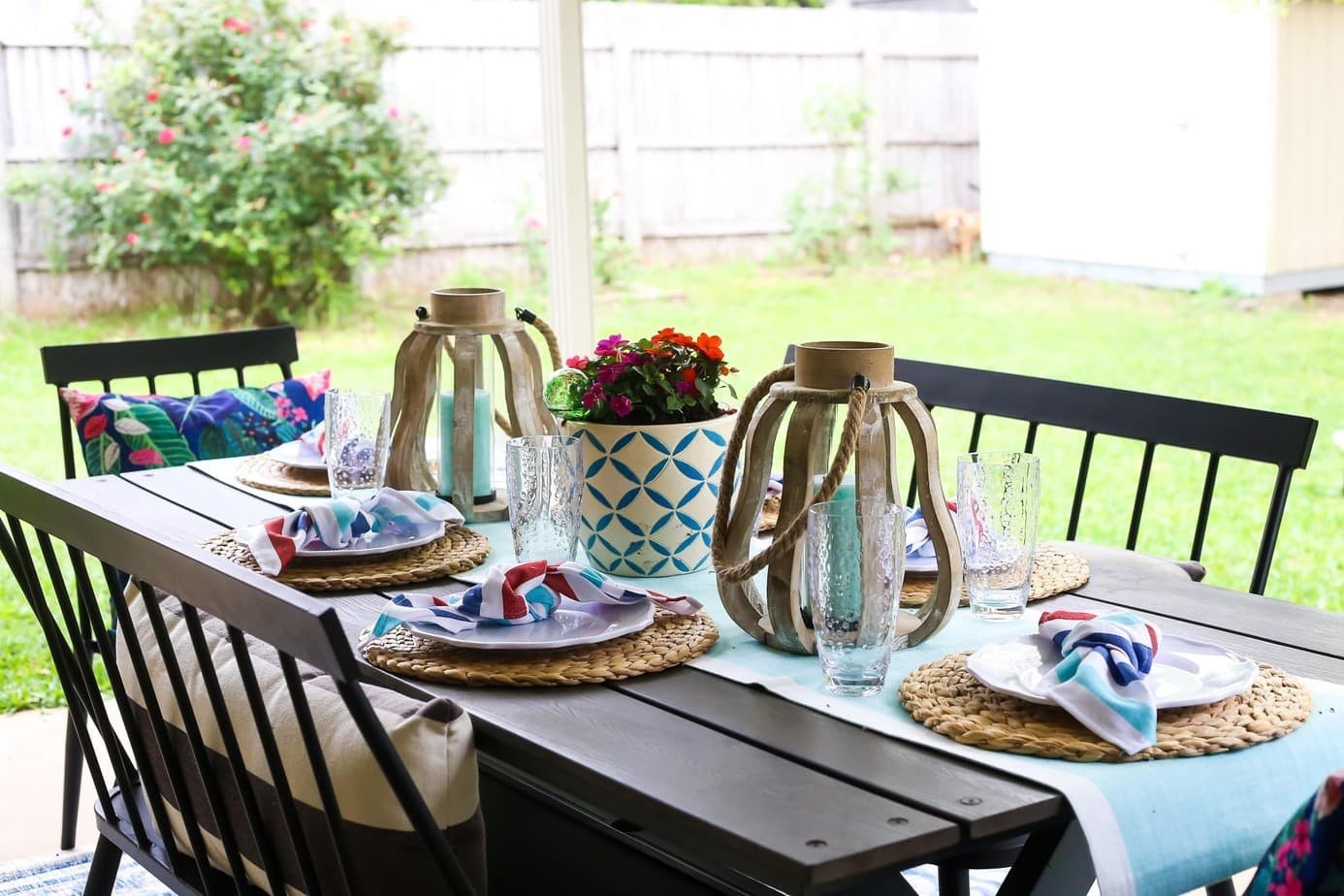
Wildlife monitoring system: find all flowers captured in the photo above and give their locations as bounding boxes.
[563,319,735,425]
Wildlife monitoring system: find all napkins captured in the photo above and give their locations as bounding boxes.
[358,560,704,646]
[230,486,465,573]
[763,470,831,499]
[1037,607,1160,752]
[905,498,985,555]
[301,415,375,468]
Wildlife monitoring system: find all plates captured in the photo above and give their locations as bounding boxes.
[265,441,328,471]
[905,557,937,576]
[233,514,447,563]
[965,633,1258,711]
[403,592,658,651]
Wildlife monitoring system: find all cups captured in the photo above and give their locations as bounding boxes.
[956,450,1042,625]
[806,499,908,698]
[320,386,393,498]
[504,434,585,566]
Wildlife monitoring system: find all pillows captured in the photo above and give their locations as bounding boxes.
[116,572,489,896]
[58,368,332,587]
[1243,769,1343,895]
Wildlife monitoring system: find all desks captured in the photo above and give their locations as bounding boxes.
[30,445,1343,896]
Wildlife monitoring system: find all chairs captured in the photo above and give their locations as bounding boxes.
[1,328,1318,895]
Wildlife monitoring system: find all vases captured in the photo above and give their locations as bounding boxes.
[562,412,728,576]
